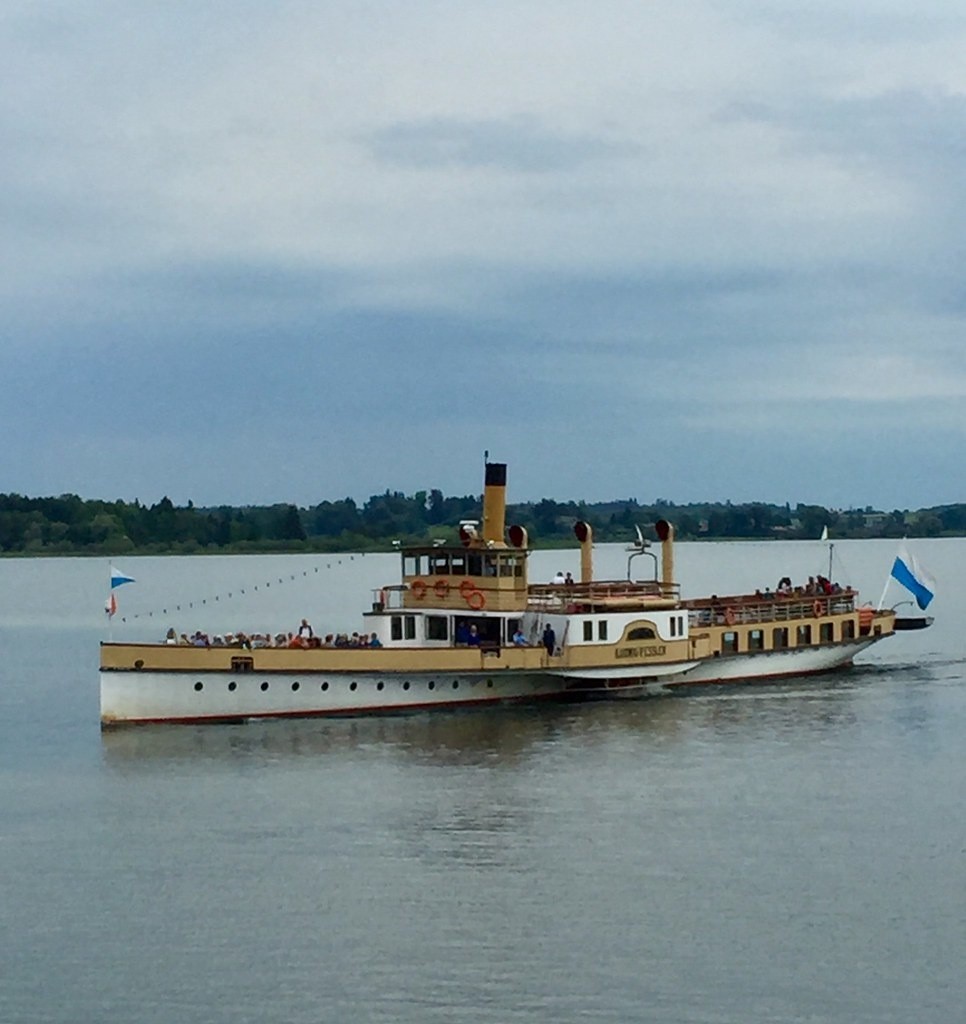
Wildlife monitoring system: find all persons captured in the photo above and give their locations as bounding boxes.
[543,623,555,656]
[166,619,384,649]
[554,572,574,584]
[711,595,721,607]
[513,628,534,648]
[756,575,843,600]
[455,619,482,648]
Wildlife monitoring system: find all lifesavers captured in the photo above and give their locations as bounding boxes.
[466,590,487,610]
[459,579,476,599]
[433,579,451,598]
[725,607,736,626]
[813,600,823,618]
[412,580,427,602]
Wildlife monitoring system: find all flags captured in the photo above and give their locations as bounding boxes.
[891,538,937,611]
[110,564,136,591]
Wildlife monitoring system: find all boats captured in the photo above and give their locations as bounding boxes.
[99,451,898,728]
